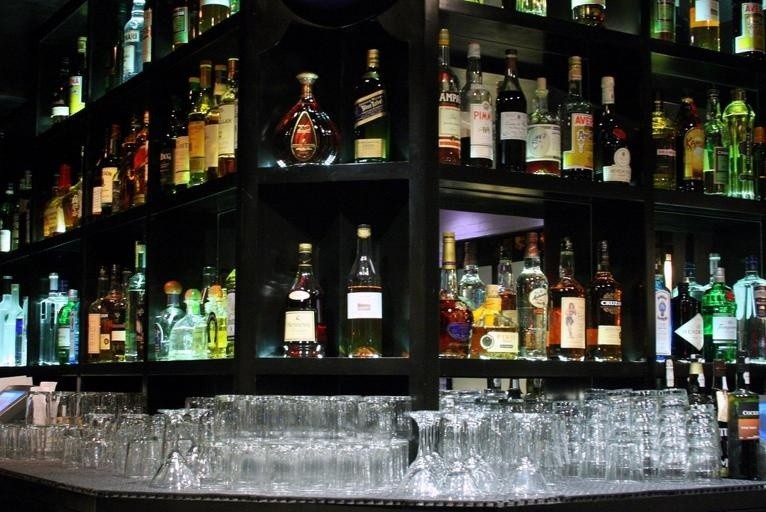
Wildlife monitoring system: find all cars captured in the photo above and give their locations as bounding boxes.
[544,384,723,480]
[206,391,416,498]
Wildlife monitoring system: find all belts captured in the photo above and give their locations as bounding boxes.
[2,388,206,489]
[405,392,552,500]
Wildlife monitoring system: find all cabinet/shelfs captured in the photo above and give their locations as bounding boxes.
[648,2,760,54]
[351,46,389,163]
[665,363,761,480]
[0,249,236,367]
[652,252,764,363]
[435,25,631,183]
[654,84,763,204]
[441,223,620,365]
[478,0,613,23]
[50,0,239,123]
[280,241,330,355]
[344,223,387,360]
[263,70,343,168]
[0,1,765,464]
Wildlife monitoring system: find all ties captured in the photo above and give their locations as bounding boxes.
[0,384,33,425]
